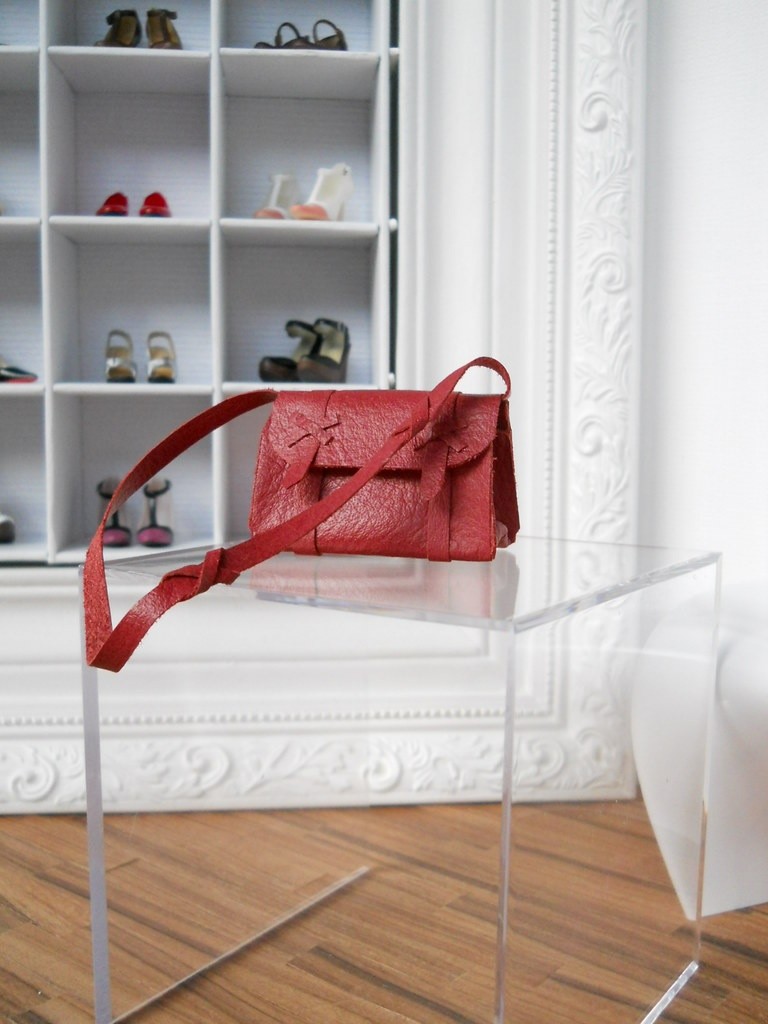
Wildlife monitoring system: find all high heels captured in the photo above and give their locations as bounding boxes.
[106,329,134,379]
[255,175,297,218]
[137,478,173,545]
[145,9,180,48]
[291,163,352,221]
[145,330,177,382]
[93,10,143,48]
[258,318,350,381]
[97,479,129,548]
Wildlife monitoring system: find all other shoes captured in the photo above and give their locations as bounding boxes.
[0,515,15,544]
[140,193,171,217]
[256,20,347,50]
[97,192,128,217]
[0,367,37,384]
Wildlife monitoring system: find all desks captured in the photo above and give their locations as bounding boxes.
[74,539,717,1022]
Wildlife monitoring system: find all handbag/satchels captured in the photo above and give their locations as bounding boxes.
[83,357,522,673]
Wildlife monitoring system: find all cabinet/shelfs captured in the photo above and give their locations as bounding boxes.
[0,0,404,566]
